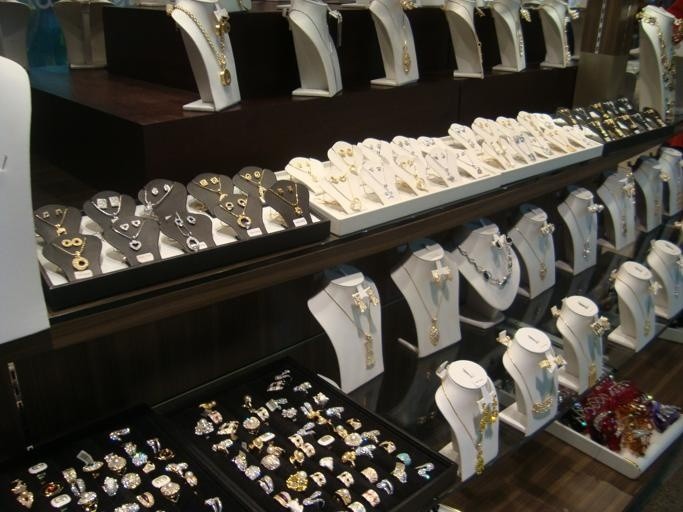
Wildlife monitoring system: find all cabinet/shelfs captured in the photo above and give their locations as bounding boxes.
[0,0,683,512]
